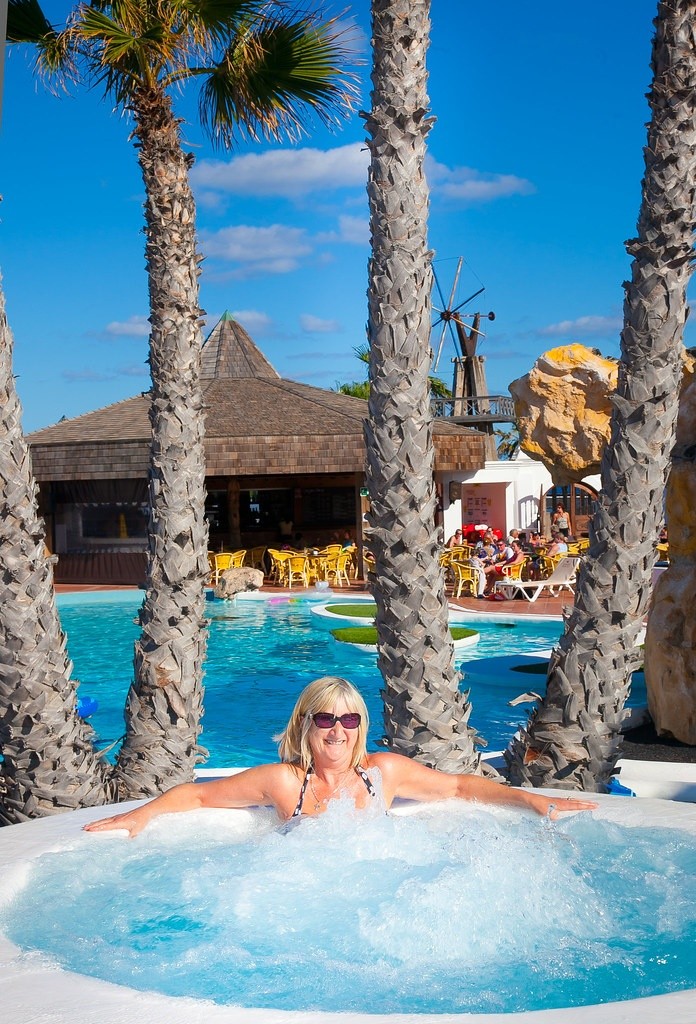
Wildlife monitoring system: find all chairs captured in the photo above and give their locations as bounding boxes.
[438,538,590,603]
[657,543,670,561]
[208,544,376,590]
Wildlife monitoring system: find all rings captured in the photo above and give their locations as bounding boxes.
[567,796,572,800]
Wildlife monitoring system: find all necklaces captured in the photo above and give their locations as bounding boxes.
[310,764,353,811]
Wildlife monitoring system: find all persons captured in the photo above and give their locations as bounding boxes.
[82,677,600,838]
[659,524,667,543]
[274,513,376,562]
[446,503,572,600]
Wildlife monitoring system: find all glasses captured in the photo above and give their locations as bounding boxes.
[301,712,362,729]
[497,544,505,546]
